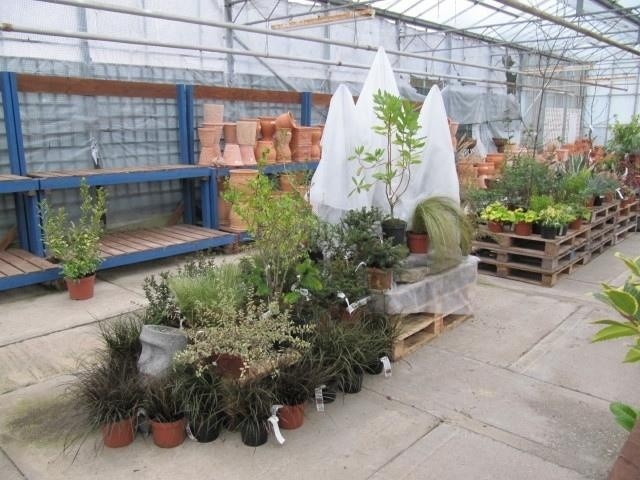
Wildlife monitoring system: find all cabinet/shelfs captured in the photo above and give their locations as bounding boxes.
[371,253,479,361]
[473,199,640,287]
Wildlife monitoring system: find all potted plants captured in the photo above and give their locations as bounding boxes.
[347,88,427,246]
[35,176,110,300]
[605,114,640,164]
[481,150,624,238]
[406,196,473,269]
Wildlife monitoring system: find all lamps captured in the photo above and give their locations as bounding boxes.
[271,9,375,32]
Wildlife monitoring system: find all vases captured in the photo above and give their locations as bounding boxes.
[450,122,610,192]
[190,97,326,233]
